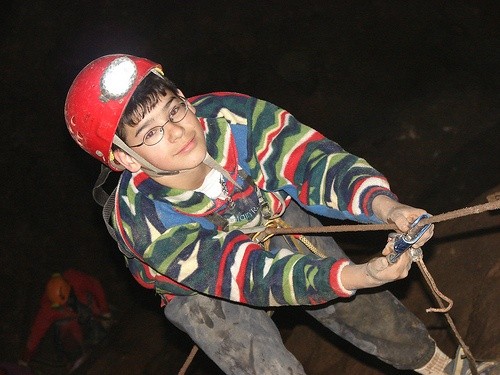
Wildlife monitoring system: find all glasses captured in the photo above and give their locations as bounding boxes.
[128,95,188,148]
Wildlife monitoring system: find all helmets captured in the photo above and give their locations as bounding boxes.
[46,275,69,305]
[64,54,163,172]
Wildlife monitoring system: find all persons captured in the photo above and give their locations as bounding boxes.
[9,270,110,373]
[66,55,499,375]
